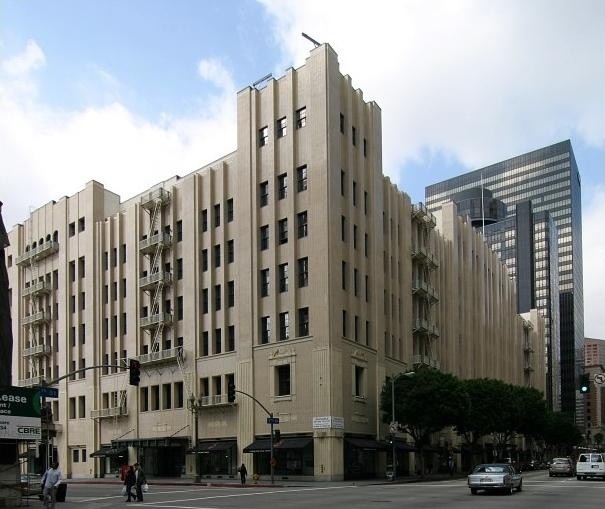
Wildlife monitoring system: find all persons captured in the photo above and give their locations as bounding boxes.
[238,463,247,487]
[133,462,147,503]
[122,465,137,502]
[38,460,62,509]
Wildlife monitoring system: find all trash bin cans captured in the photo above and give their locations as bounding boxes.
[56,484,67,502]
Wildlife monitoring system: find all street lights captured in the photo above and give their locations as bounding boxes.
[392,372,416,481]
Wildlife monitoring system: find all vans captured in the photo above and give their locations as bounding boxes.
[576,453,605,480]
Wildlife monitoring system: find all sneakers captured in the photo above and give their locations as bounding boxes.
[134,496,136,500]
[125,500,131,502]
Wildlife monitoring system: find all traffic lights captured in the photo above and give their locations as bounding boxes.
[228,382,235,402]
[130,359,140,386]
[579,372,590,394]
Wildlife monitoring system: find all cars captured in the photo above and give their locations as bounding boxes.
[21,473,42,496]
[549,460,573,477]
[468,463,522,495]
